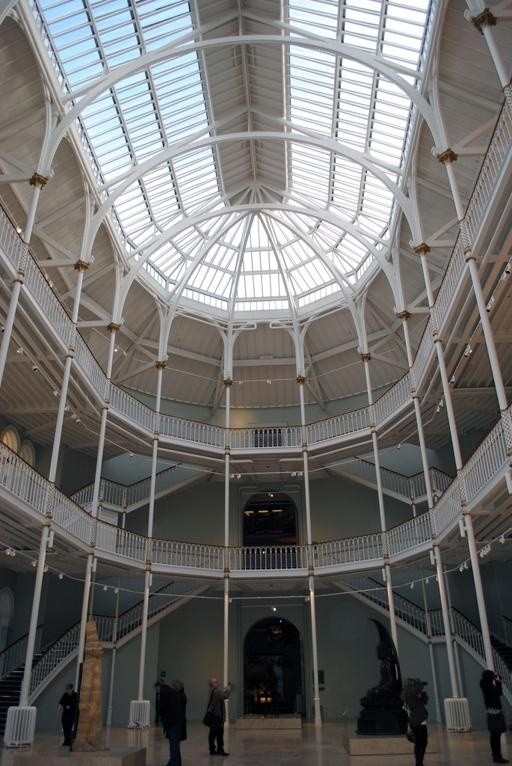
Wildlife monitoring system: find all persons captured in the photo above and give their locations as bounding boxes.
[402,679,428,766]
[156,681,169,735]
[480,670,509,763]
[57,683,79,746]
[208,677,232,756]
[160,680,187,766]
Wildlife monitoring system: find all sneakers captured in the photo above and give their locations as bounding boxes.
[492,757,511,764]
[209,750,230,757]
[62,741,73,747]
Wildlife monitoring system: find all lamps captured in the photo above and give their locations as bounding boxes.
[0,257,510,604]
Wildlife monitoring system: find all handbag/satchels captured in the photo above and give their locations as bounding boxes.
[202,710,212,728]
[407,729,417,743]
